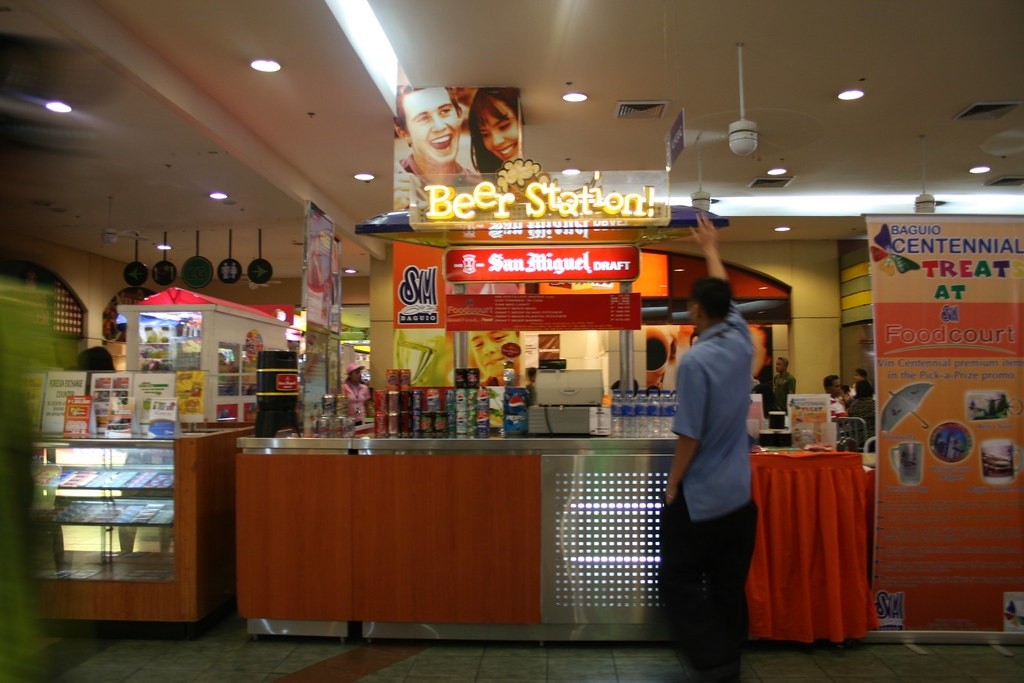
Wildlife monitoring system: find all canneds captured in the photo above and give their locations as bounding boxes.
[373,368,490,434]
[503,385,531,435]
[317,394,355,438]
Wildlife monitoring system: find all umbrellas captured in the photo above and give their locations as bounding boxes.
[880,382,934,433]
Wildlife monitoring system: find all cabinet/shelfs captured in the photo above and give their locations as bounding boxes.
[42,439,179,581]
[138,342,171,363]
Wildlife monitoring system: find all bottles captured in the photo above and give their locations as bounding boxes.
[610,389,623,437]
[635,390,649,438]
[649,390,660,438]
[672,391,678,438]
[660,390,672,438]
[623,390,635,437]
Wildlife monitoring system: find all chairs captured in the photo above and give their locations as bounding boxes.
[836,416,868,453]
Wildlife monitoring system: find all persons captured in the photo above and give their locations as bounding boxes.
[824,368,876,418]
[774,357,796,412]
[340,362,375,426]
[469,329,522,386]
[73,345,195,553]
[392,85,523,210]
[690,325,773,418]
[660,213,756,683]
[524,367,538,402]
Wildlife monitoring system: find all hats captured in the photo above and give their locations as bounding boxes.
[346,364,365,375]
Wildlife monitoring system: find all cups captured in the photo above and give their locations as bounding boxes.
[979,439,1024,485]
[889,442,922,485]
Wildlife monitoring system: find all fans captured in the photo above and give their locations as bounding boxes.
[913,135,947,213]
[670,40,830,212]
